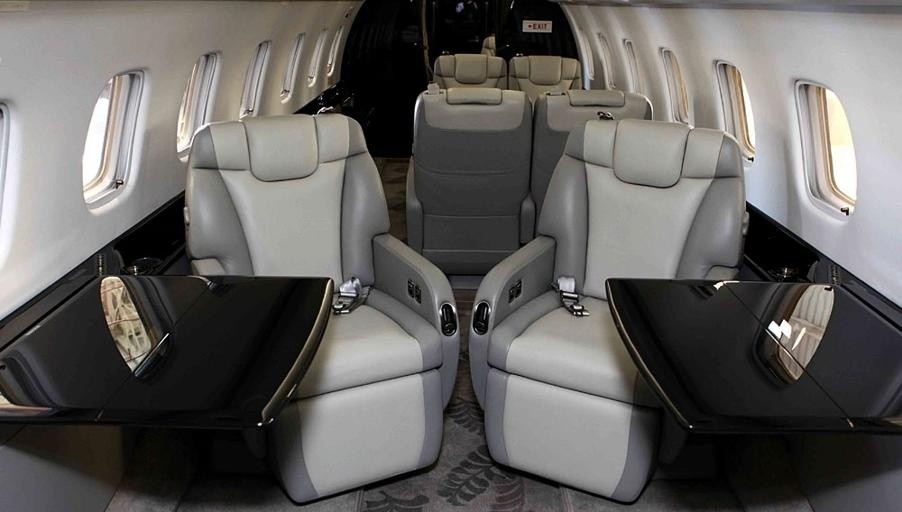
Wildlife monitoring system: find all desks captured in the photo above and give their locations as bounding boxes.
[602,274,900,437]
[8,272,335,428]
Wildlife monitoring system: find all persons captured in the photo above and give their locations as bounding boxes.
[372,28,419,158]
